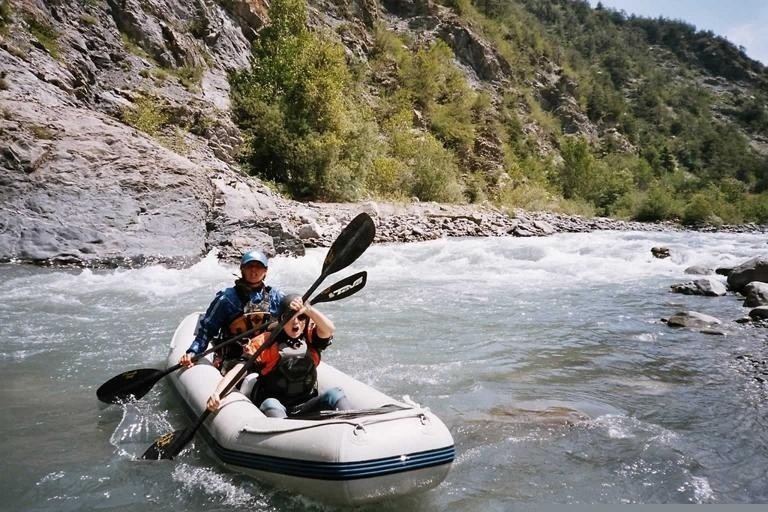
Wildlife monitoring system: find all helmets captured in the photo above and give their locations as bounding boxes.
[277,295,310,321]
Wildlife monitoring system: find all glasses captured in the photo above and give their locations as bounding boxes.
[281,312,306,321]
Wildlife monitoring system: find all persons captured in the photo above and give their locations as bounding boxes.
[205,291,355,420]
[176,249,292,372]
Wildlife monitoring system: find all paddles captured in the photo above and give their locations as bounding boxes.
[96,271,367,405]
[140,212,376,460]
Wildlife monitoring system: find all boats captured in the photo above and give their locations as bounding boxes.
[166,311,457,506]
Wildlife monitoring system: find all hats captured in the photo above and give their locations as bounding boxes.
[240,249,268,268]
